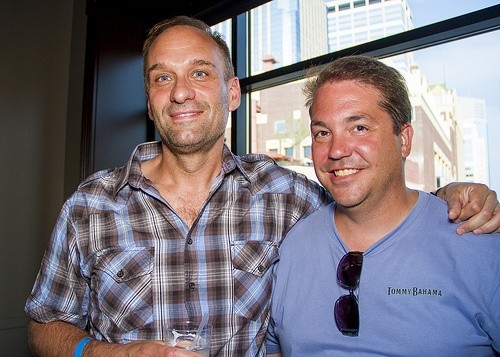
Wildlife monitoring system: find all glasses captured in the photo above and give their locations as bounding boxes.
[333,251,363,337]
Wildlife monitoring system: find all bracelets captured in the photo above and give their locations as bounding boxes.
[73,337,95,356]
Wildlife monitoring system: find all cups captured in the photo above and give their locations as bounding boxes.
[163,322,212,356]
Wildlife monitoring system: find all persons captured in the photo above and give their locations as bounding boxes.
[266,51,500,357]
[21,16,500,357]
